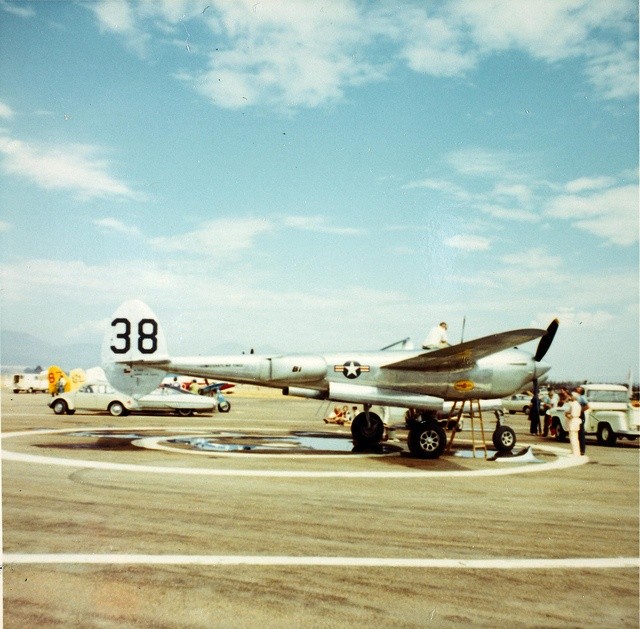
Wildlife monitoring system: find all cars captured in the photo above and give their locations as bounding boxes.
[133,385,218,415]
[48,380,138,416]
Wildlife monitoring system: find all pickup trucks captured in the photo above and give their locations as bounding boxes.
[501,394,545,415]
[546,383,640,446]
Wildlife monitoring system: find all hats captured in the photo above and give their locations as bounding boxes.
[571,391,580,400]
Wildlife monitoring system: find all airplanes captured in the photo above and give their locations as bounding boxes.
[99,298,560,461]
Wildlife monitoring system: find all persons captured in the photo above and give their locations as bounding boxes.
[528,390,546,435]
[166,376,180,388]
[187,379,199,393]
[349,404,360,424]
[574,385,590,453]
[323,406,345,424]
[336,406,351,426]
[577,386,585,395]
[56,376,65,393]
[51,392,58,397]
[560,394,582,455]
[541,387,560,437]
[555,387,569,407]
[421,321,449,351]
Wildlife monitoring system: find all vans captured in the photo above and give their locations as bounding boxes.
[10,374,49,393]
